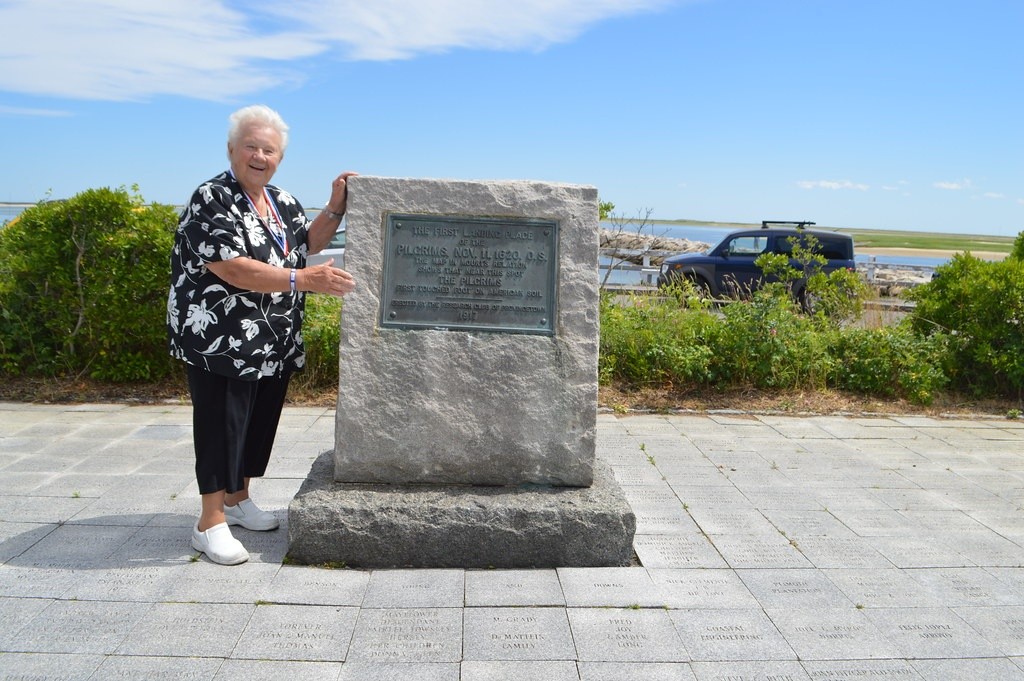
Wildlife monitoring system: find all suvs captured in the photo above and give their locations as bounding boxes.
[656,220,855,315]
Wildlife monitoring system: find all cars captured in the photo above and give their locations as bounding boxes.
[304,229,346,295]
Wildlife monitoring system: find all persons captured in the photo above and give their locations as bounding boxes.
[164,107,359,566]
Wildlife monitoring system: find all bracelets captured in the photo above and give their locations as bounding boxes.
[288,268,296,290]
[323,206,345,220]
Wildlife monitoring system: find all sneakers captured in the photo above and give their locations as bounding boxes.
[191,518,249,564]
[224,498,280,530]
